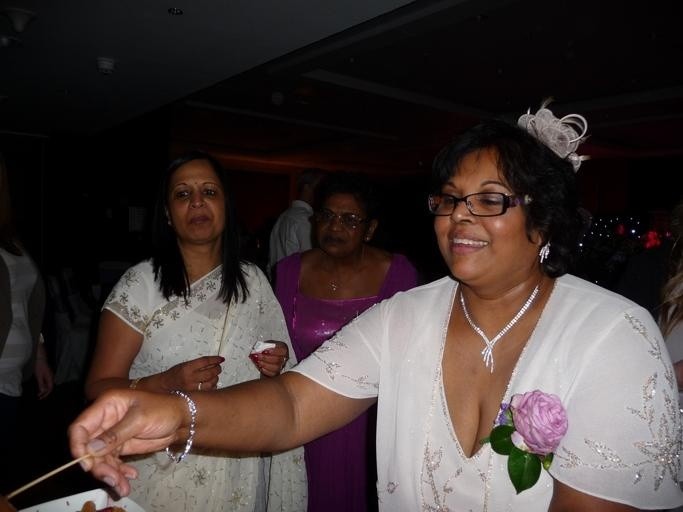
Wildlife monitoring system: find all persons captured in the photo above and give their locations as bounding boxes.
[81,148,309,512]
[265,177,419,512]
[66,109,683,512]
[262,163,683,395]
[0,173,53,485]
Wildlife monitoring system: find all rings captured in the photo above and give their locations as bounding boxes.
[198,382,202,392]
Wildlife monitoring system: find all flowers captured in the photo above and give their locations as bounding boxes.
[481,390,568,494]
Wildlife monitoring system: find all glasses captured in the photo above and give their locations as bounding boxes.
[315,208,367,231]
[426,191,534,217]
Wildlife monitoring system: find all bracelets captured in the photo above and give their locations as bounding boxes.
[128,376,143,391]
[165,390,198,464]
[35,355,47,362]
